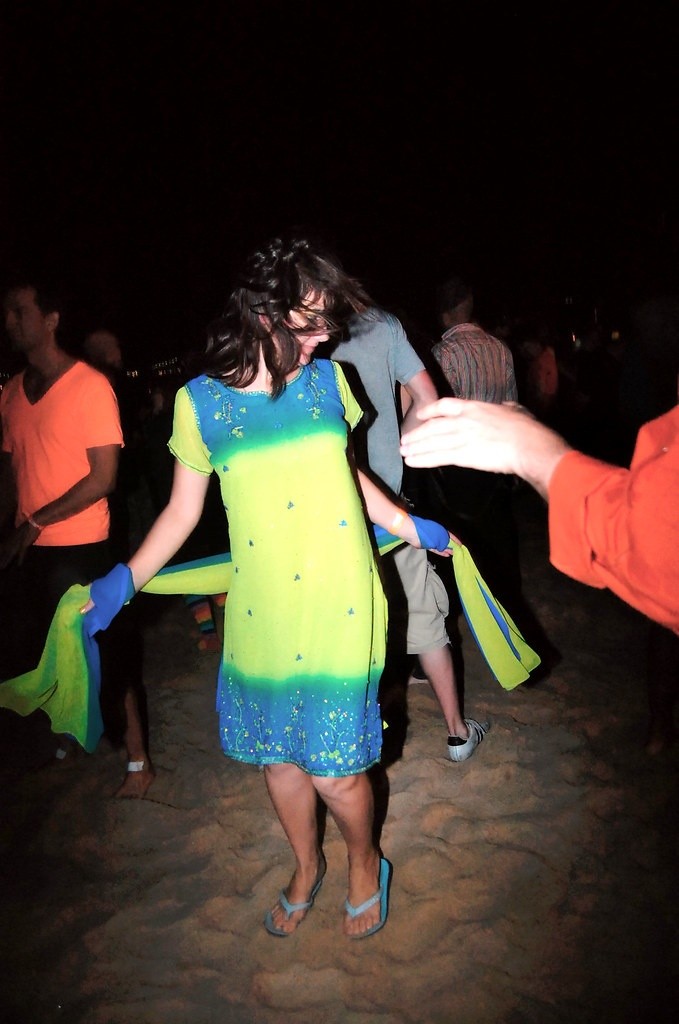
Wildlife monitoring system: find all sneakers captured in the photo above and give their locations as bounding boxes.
[447,718,490,761]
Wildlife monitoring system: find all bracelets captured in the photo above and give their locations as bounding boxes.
[27,514,43,529]
[388,509,408,535]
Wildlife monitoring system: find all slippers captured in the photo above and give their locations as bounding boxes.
[342,857,390,940]
[264,880,322,936]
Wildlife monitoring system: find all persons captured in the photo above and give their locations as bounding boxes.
[310,289,492,763]
[401,396,679,635]
[73,324,210,442]
[0,277,153,800]
[383,273,623,521]
[0,224,540,938]
